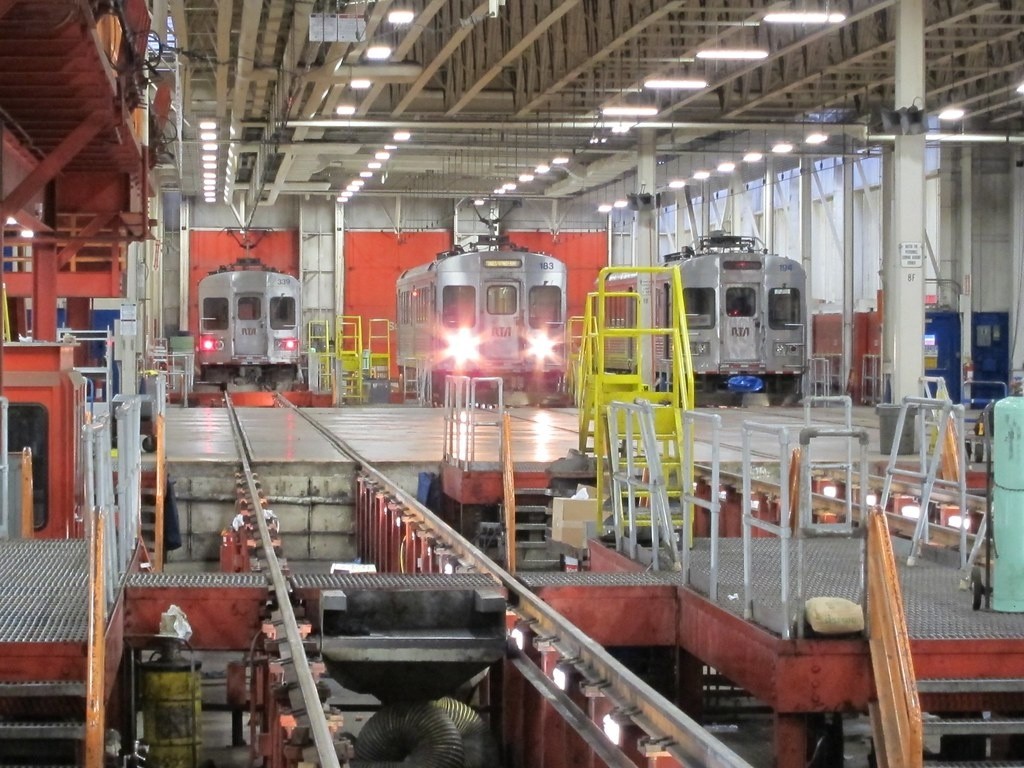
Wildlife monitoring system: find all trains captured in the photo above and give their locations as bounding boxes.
[197,263,302,392]
[592,231,808,395]
[395,236,568,406]
